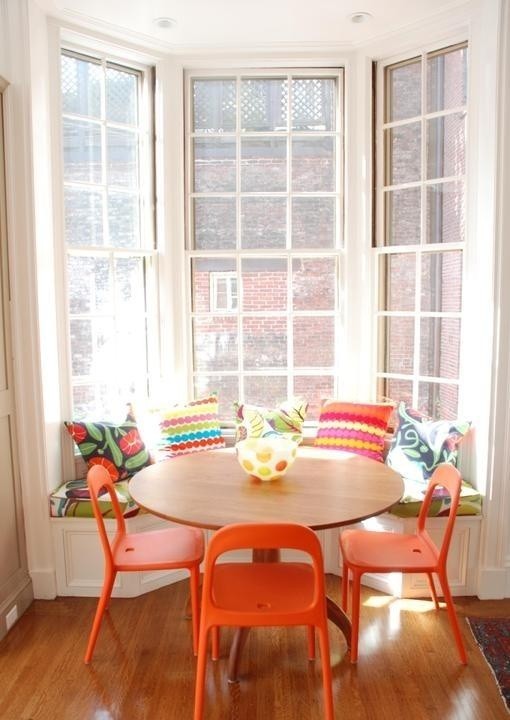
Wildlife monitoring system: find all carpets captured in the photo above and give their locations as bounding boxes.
[465,616,510,715]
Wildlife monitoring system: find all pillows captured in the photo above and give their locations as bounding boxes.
[384,400,475,502]
[63,401,155,483]
[155,389,225,461]
[312,394,398,463]
[231,396,308,446]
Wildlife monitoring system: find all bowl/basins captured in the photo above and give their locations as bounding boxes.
[235,436,298,483]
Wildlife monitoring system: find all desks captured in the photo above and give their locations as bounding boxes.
[128,447,405,683]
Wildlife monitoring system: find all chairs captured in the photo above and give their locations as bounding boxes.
[83,464,200,665]
[192,522,334,720]
[338,462,469,666]
